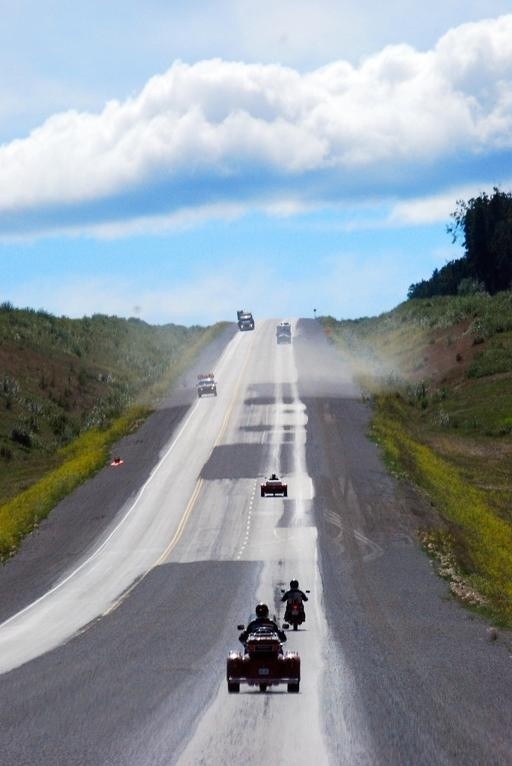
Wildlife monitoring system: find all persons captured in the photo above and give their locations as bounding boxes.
[281,579,309,621]
[270,473,279,480]
[238,603,287,647]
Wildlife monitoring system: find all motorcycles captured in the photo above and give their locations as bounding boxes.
[280,588,313,631]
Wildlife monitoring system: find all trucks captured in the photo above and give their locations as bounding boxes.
[276,322,292,344]
[237,310,254,331]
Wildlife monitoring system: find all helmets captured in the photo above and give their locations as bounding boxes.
[255,604,269,617]
[290,580,298,589]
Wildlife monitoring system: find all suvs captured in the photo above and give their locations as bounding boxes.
[196,373,220,397]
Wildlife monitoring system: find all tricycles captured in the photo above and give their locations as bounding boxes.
[260,472,288,497]
[221,617,305,695]
